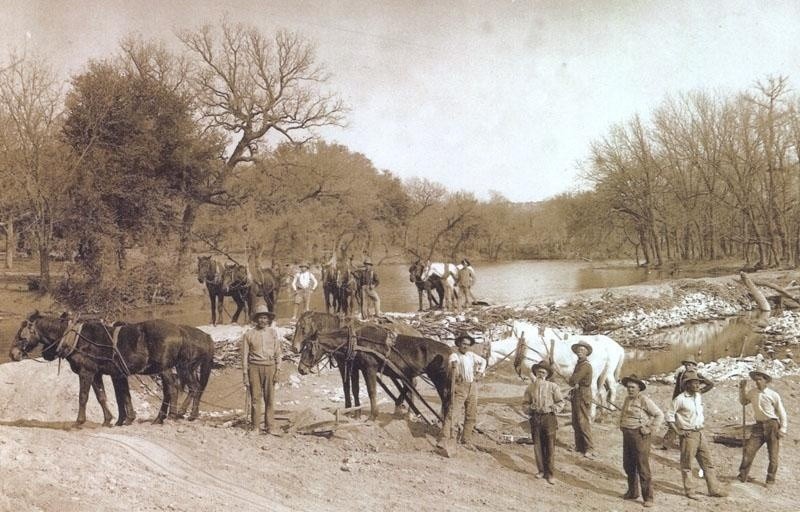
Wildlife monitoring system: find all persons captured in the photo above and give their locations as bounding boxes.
[660,354,715,450]
[569,340,593,458]
[358,257,384,321]
[521,360,564,486]
[289,262,319,320]
[239,304,282,438]
[438,331,487,451]
[453,258,476,307]
[667,371,729,499]
[737,366,789,487]
[618,374,664,507]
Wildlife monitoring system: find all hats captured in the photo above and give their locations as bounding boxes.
[682,370,705,382]
[455,333,475,347]
[572,341,592,356]
[249,304,275,322]
[532,361,554,378]
[363,259,373,266]
[681,354,697,367]
[299,262,310,270]
[749,368,772,383]
[621,374,646,391]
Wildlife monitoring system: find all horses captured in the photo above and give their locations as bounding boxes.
[320,263,352,314]
[196,255,253,326]
[290,308,426,419]
[408,261,445,311]
[7,307,194,428]
[333,264,374,312]
[503,317,627,414]
[219,261,278,328]
[40,311,215,429]
[296,325,459,439]
[421,260,476,311]
[477,333,608,423]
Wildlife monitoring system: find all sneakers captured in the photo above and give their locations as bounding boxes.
[460,437,477,452]
[622,491,638,500]
[708,490,729,497]
[584,451,593,458]
[436,437,445,449]
[643,501,653,507]
[546,477,555,485]
[535,471,544,478]
